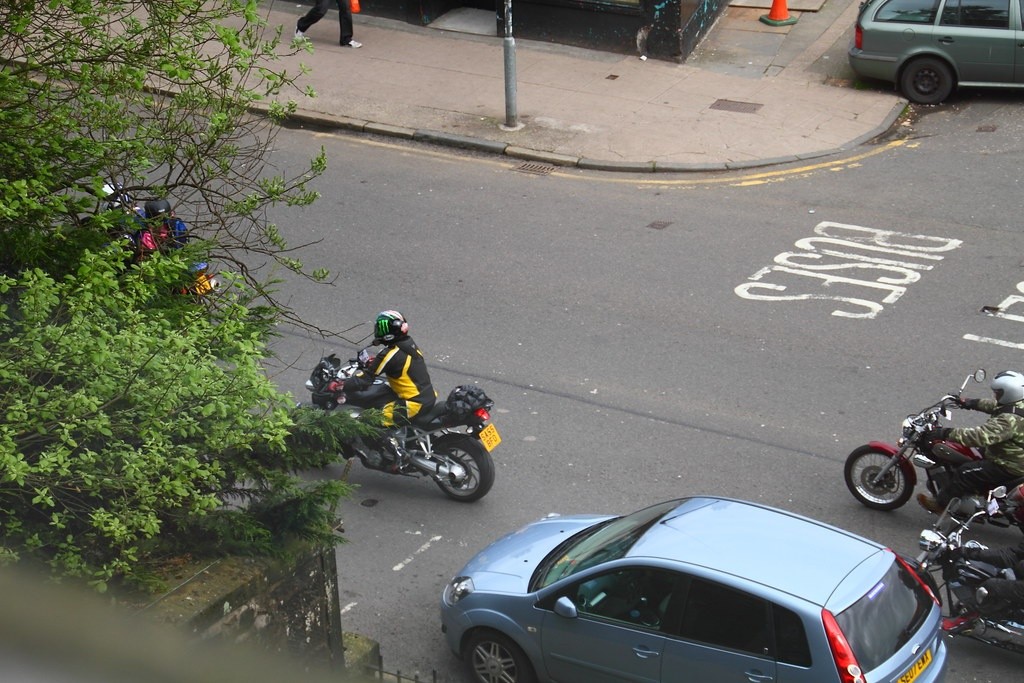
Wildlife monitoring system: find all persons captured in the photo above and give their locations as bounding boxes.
[951,483,1024,618]
[100,181,189,265]
[916,370,1024,516]
[325,310,437,471]
[294,0,362,48]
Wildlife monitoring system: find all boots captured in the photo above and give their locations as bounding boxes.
[384,437,410,471]
[942,599,986,636]
[917,488,953,517]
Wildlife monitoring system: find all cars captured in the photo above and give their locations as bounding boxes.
[847,0,1024,105]
[440,495,948,683]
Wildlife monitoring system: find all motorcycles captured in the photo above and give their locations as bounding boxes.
[295,350,502,504]
[101,183,221,323]
[843,368,1024,534]
[913,485,1024,654]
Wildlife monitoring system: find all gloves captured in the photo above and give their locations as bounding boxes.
[327,381,344,391]
[961,398,980,410]
[950,546,967,564]
[369,355,377,366]
[927,427,951,440]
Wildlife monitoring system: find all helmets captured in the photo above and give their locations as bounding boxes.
[373,310,408,346]
[143,197,171,225]
[1006,483,1024,525]
[100,181,128,209]
[991,370,1024,406]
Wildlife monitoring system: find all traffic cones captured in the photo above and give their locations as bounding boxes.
[759,0,798,26]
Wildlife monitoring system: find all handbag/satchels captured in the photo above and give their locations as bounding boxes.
[349,0,360,13]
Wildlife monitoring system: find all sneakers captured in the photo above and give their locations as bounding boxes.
[294,21,304,44]
[342,39,362,49]
